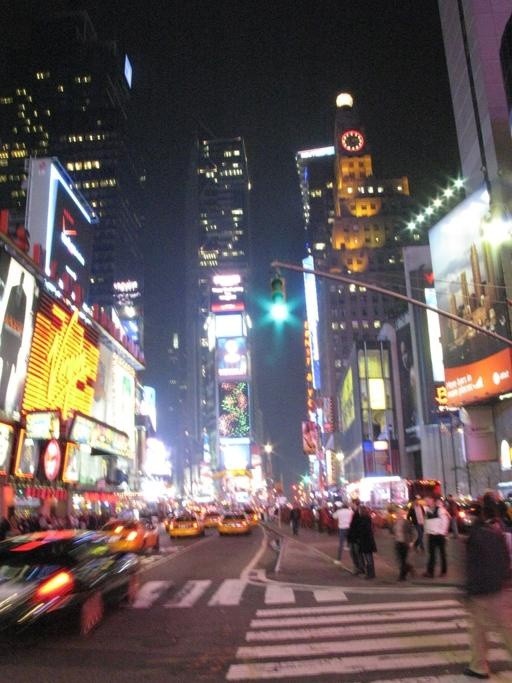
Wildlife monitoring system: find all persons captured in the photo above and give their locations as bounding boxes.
[455,489,511,680]
[0,503,175,541]
[260,492,511,581]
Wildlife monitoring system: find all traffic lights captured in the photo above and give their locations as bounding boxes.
[269,276,287,324]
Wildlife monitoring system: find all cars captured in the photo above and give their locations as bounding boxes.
[0,498,261,640]
[383,494,479,538]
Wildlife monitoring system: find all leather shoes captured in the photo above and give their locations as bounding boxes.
[462,666,489,679]
[333,558,449,582]
